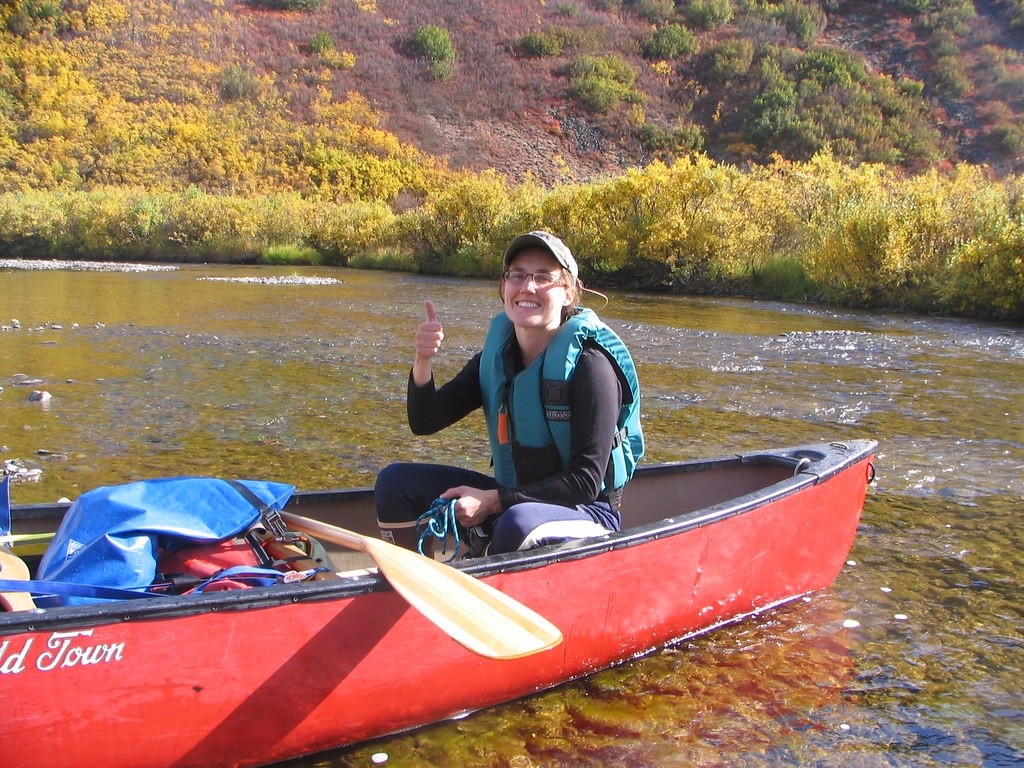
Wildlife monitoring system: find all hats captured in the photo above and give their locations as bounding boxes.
[502,230,579,278]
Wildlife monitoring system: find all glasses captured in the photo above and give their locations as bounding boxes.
[504,270,573,290]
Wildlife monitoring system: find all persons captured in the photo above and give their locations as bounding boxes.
[377,230,646,560]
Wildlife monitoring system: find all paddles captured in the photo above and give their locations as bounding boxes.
[276,512,563,661]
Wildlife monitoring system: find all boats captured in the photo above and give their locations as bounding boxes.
[0,438,881,768]
